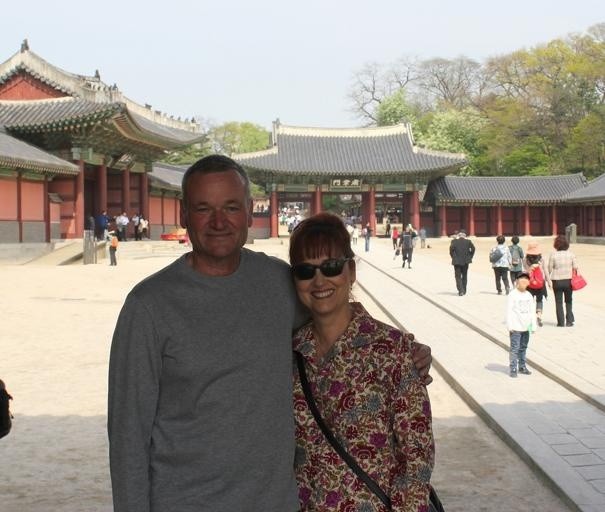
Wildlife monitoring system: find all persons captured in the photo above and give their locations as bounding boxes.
[525,242,552,326]
[88,210,150,266]
[509,237,525,286]
[548,234,576,327]
[507,273,536,378]
[491,235,512,296]
[289,212,433,512]
[256,203,429,270]
[107,156,433,512]
[450,228,475,296]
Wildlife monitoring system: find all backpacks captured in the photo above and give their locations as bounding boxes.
[527,263,544,289]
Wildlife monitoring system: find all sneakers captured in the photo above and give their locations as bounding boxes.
[566,322,573,327]
[537,316,545,327]
[557,322,565,327]
[510,368,517,377]
[519,367,531,374]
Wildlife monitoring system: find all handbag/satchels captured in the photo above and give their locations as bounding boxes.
[571,276,586,290]
[490,246,503,263]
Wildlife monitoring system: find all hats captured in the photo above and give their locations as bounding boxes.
[524,241,541,255]
[517,272,529,278]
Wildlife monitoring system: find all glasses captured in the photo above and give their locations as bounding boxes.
[288,258,351,280]
